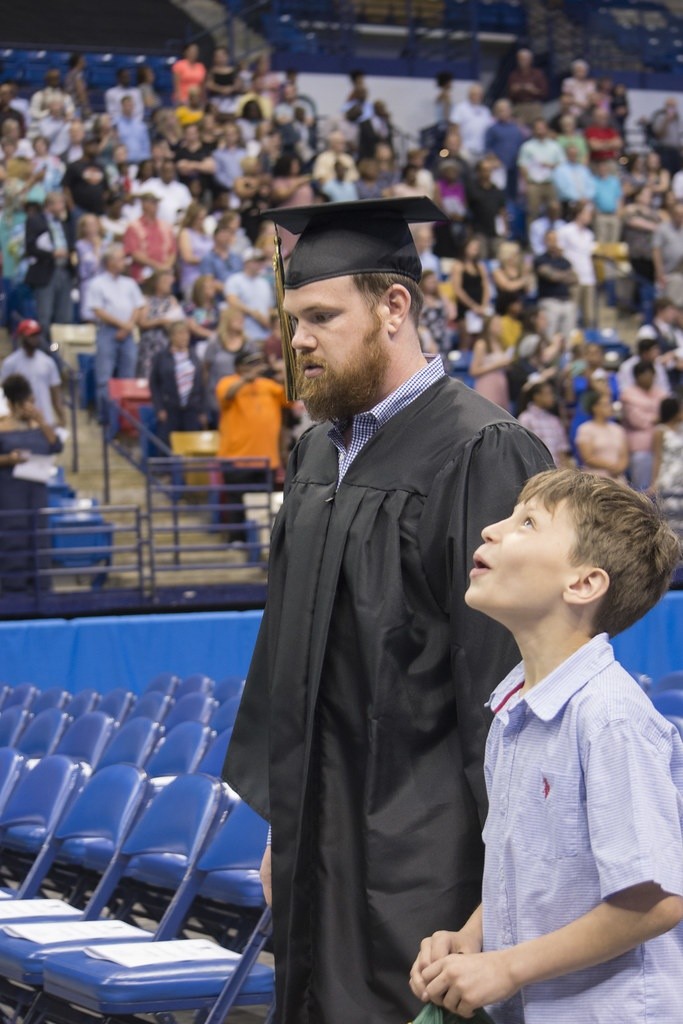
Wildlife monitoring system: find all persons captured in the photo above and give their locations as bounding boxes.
[415,459,683,1023]
[221,195,557,1024]
[0,47,683,493]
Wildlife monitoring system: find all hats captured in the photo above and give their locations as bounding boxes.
[258,193,449,405]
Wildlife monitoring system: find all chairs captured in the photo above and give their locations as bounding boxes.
[0,672,683,1024]
